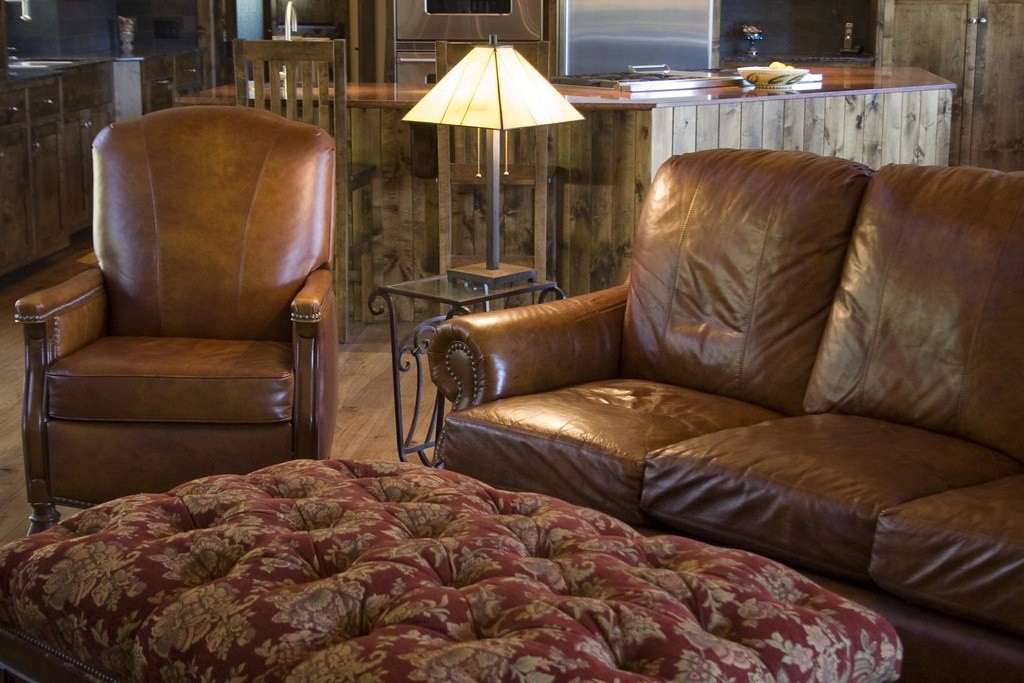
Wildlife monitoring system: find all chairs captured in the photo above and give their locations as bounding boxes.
[434,41,551,324]
[233,39,350,345]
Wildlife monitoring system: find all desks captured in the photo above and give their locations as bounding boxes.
[174,64,956,323]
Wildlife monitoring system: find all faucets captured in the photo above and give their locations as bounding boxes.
[279,0,298,100]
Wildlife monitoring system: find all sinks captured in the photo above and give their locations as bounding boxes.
[262,79,336,89]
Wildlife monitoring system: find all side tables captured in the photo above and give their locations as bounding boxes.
[367,275,565,469]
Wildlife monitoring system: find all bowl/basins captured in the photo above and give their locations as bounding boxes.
[737,66,810,90]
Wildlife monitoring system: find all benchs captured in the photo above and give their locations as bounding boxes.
[0,457,903,683]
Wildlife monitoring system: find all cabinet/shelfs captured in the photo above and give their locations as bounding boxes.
[114,53,199,116]
[58,62,116,237]
[0,76,71,277]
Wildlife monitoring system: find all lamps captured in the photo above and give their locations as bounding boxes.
[401,35,587,290]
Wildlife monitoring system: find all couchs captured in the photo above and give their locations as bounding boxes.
[426,149,1024,683]
[15,106,339,536]
